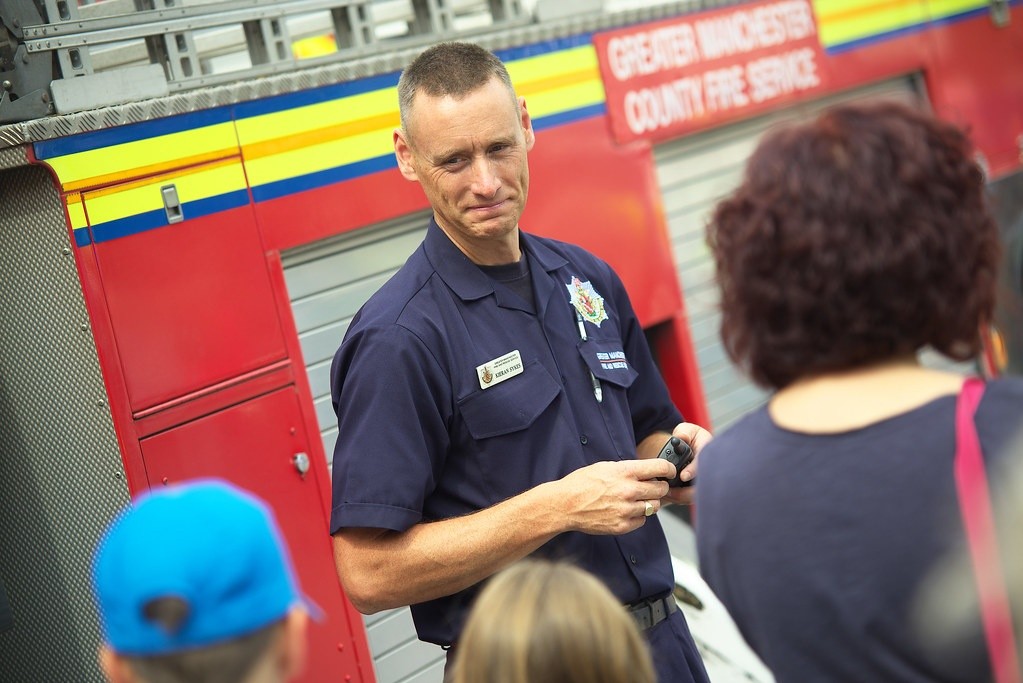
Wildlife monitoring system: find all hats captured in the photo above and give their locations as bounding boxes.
[94,479,298,657]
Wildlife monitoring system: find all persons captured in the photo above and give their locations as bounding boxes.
[446,558,657,682]
[328,41,713,682]
[691,92,1022,682]
[91,476,326,683]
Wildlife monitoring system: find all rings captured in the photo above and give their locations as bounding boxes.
[644,500,653,516]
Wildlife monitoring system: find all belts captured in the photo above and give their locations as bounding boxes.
[624,591,680,631]
